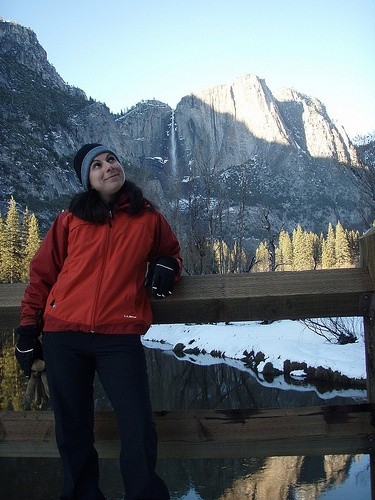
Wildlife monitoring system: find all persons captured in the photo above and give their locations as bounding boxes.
[13,144,185,500]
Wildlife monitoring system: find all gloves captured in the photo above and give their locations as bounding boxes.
[147,256,179,299]
[14,325,43,379]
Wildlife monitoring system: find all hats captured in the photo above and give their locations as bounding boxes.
[74,143,122,193]
